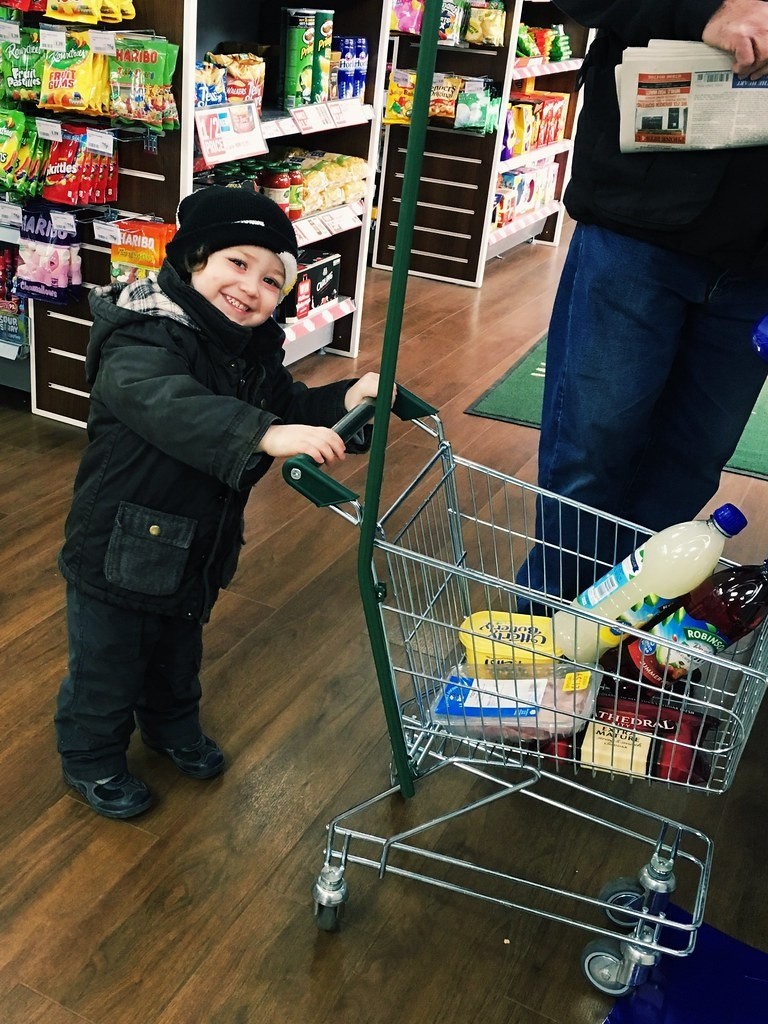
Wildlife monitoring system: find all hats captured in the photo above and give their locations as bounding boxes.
[164,186,297,306]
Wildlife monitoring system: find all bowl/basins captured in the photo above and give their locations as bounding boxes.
[425,663,604,743]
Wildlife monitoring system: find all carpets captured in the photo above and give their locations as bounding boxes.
[462,330,768,479]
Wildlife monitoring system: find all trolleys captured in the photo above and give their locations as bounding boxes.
[280,380,768,996]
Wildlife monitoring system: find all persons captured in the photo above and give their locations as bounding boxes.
[49,185,397,821]
[513,0,768,618]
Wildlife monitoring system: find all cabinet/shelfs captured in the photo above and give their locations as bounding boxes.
[0,0,397,426]
[368,0,593,287]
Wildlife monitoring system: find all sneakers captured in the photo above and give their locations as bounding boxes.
[161,735,225,778]
[61,760,156,819]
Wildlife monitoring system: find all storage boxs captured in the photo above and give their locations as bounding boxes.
[426,663,608,734]
[457,612,566,679]
[274,251,340,322]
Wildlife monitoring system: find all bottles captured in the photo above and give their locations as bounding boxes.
[598,559,768,698]
[548,503,748,664]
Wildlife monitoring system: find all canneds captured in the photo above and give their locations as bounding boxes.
[212,160,303,222]
